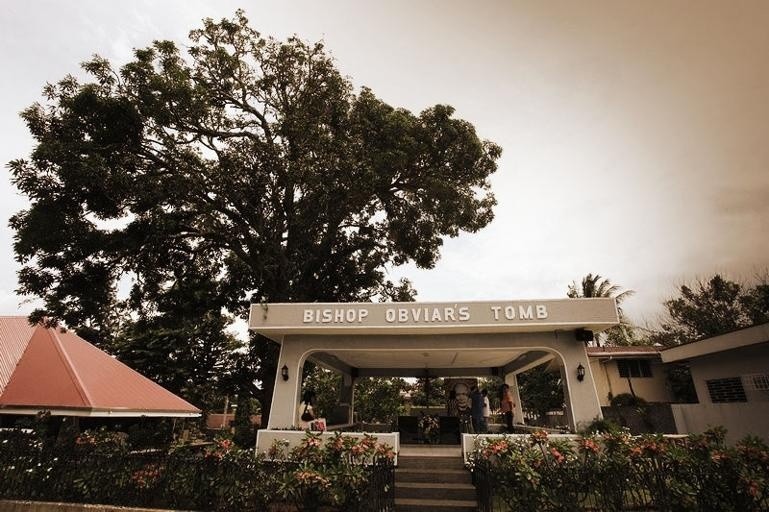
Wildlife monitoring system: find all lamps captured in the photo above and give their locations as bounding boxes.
[576,361,586,382]
[281,362,289,381]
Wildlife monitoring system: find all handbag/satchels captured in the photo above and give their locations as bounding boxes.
[301,404,315,421]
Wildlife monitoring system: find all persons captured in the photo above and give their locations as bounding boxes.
[446,382,472,418]
[296,390,328,432]
[480,388,492,433]
[498,383,517,434]
[467,385,483,434]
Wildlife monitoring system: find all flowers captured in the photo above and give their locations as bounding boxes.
[419,414,442,439]
